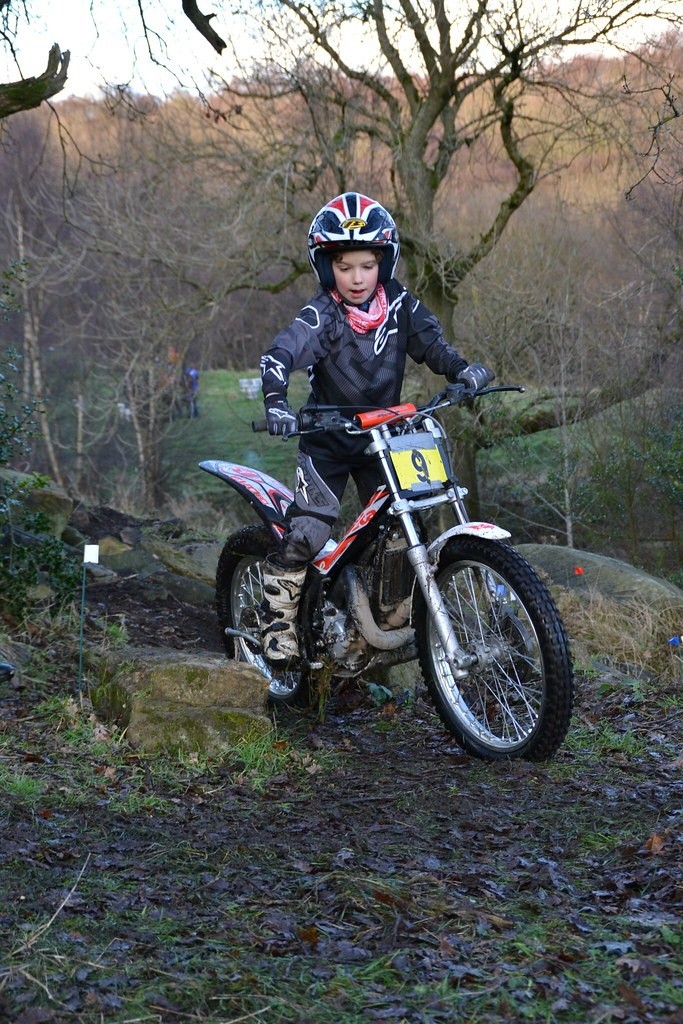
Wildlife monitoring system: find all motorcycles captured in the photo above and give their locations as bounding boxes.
[196,369,576,765]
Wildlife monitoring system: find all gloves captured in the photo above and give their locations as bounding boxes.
[457,362,490,391]
[263,394,300,436]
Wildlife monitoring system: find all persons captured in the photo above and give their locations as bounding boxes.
[260,193,497,662]
[181,367,199,418]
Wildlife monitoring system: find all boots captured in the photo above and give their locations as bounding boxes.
[261,553,308,666]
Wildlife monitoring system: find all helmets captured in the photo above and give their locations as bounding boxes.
[307,192,400,284]
